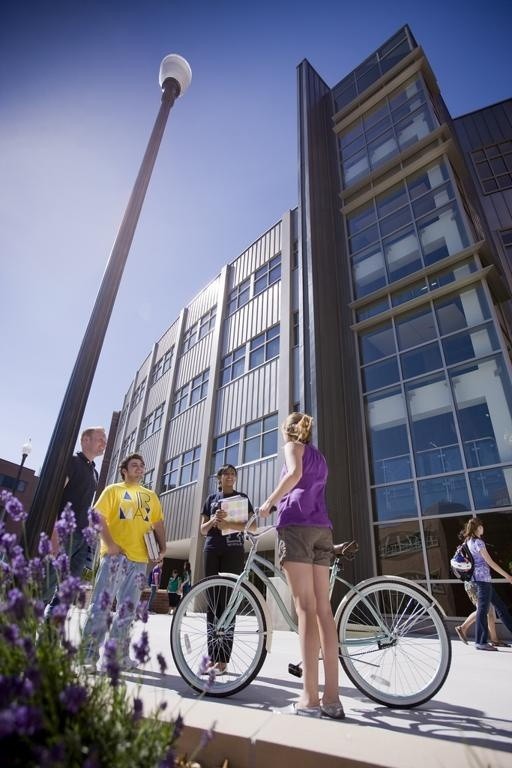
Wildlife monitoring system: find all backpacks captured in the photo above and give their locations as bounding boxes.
[147,570,153,587]
[450,540,475,583]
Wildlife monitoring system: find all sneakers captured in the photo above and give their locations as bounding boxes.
[491,640,512,648]
[454,626,468,645]
[77,664,97,675]
[168,611,174,615]
[204,665,228,676]
[319,697,346,720]
[147,609,156,615]
[474,643,498,651]
[118,657,138,671]
[272,702,322,718]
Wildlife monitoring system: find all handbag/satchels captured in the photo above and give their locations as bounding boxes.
[182,585,191,595]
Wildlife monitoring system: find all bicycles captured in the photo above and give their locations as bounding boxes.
[170,505,452,709]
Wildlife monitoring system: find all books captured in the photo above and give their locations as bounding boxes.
[148,530,159,559]
[217,494,248,537]
[143,533,154,560]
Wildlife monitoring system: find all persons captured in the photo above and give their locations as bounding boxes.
[166,569,181,615]
[462,516,512,651]
[258,411,345,722]
[195,464,257,675]
[178,562,190,616]
[36,425,108,657]
[75,454,168,677]
[453,527,509,648]
[145,560,163,616]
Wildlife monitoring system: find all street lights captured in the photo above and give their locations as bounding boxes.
[20,50,199,588]
[2,437,32,525]
